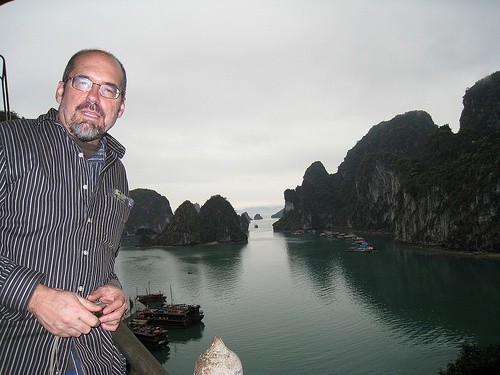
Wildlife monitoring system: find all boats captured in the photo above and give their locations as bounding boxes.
[320,232,372,253]
[124,291,204,347]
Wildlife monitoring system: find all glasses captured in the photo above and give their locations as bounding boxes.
[63,77,124,100]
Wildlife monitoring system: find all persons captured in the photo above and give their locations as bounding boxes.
[0,49,134,374]
